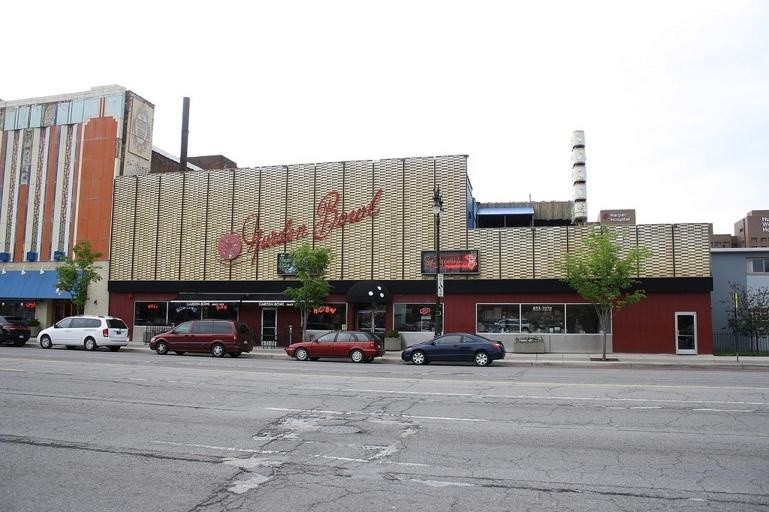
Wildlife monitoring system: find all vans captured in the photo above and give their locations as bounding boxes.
[149,318,254,357]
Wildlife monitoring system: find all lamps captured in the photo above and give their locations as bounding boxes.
[71,288,78,305]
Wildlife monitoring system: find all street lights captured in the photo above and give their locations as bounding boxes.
[431,183,445,339]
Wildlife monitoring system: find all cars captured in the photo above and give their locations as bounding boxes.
[135,311,180,325]
[361,318,589,334]
[400,330,506,367]
[285,329,386,364]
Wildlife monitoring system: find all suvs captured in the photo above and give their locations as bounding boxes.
[0,315,31,347]
[35,314,130,352]
